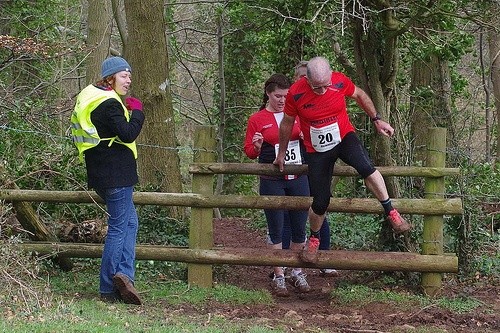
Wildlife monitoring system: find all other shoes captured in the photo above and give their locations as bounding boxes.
[100,293,124,304]
[114,274,142,305]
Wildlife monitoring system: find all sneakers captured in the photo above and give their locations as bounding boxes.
[300,235,320,263]
[320,269,338,277]
[387,209,410,232]
[269,273,289,296]
[290,270,311,292]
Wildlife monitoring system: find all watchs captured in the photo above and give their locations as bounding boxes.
[371,113,381,121]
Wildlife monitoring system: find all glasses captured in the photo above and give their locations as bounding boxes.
[309,77,333,91]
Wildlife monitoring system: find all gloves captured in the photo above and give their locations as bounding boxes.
[125,97,142,111]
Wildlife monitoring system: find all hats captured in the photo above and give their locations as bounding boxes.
[101,56,132,78]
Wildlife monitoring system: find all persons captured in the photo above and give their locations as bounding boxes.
[273,56,412,266]
[244,73,311,297]
[294,60,338,277]
[70,57,145,305]
